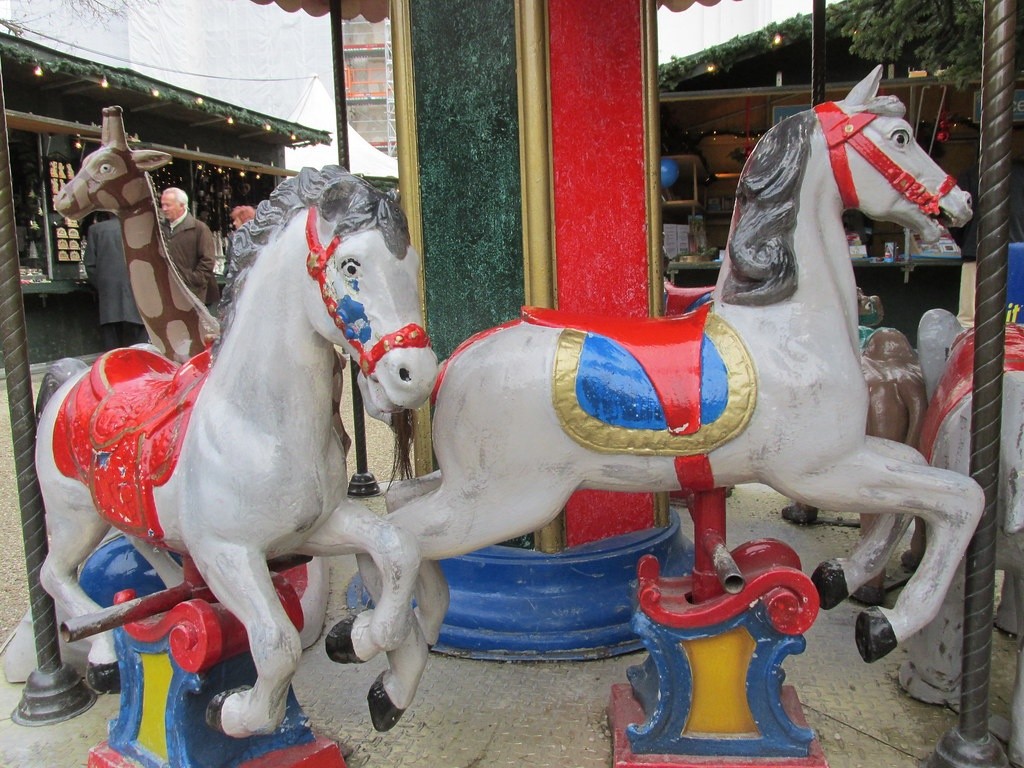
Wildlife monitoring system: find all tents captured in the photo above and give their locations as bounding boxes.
[284,76,398,177]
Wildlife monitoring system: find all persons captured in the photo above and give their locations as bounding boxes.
[83,213,150,351]
[161,187,220,305]
[222,205,255,277]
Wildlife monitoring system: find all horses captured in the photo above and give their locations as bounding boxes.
[32,72,985,741]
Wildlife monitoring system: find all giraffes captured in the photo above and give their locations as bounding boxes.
[54,104,225,378]
